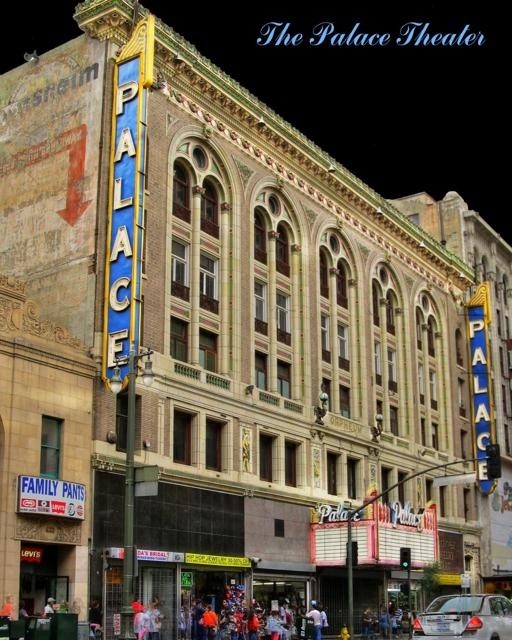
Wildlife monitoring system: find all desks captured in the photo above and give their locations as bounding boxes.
[110,347,155,639]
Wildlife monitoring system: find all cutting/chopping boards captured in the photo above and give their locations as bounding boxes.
[340,624,350,640]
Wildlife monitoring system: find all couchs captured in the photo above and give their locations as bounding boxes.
[400,548,411,570]
[487,444,501,480]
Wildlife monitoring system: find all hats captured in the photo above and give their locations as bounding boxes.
[411,593,512,640]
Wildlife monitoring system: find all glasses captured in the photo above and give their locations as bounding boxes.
[370,406,383,444]
[24,50,40,64]
[328,164,335,171]
[459,272,464,278]
[419,240,425,247]
[376,206,382,214]
[257,115,265,127]
[314,383,329,425]
